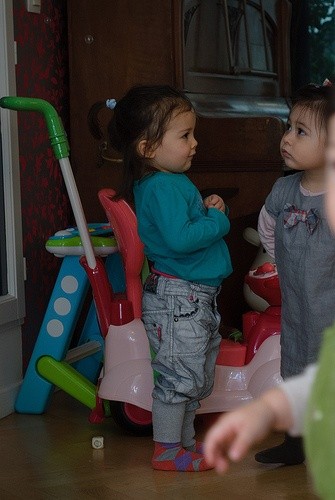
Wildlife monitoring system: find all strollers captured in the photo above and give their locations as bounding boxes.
[0,97,282,436]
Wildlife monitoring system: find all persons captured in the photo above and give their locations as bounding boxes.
[202,116,335,499]
[254,77,335,465]
[87,82,234,471]
